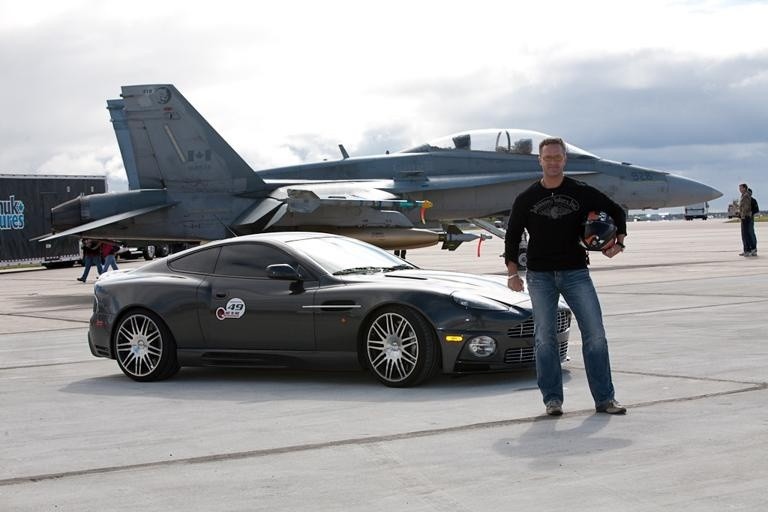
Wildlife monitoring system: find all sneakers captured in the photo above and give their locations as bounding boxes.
[596,402,626,413]
[77,277,86,283]
[738,247,759,257]
[546,399,563,416]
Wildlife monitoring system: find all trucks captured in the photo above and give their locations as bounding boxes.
[728,198,741,219]
[684,202,709,221]
[0,174,107,269]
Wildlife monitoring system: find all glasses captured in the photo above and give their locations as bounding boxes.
[538,155,566,160]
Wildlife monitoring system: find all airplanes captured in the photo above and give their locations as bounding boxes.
[29,84,724,251]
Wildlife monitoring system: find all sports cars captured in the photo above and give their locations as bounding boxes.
[88,232,572,388]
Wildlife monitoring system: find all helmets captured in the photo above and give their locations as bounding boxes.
[582,210,616,250]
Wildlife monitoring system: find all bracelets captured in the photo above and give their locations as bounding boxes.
[616,241,625,249]
[507,273,519,280]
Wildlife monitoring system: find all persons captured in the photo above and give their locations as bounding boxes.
[502,136,628,418]
[393,247,407,259]
[74,239,102,284]
[738,183,755,258]
[746,187,759,258]
[95,240,119,279]
